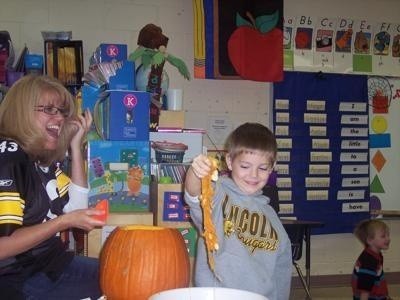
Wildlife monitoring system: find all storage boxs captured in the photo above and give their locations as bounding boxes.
[149,131,203,163]
[89,43,127,65]
[68,140,151,213]
[92,90,150,141]
[158,109,184,129]
[104,61,135,91]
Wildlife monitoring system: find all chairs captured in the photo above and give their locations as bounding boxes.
[283,224,312,300]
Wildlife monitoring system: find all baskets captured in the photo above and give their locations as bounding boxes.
[154,148,185,163]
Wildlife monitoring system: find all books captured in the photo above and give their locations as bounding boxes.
[45,42,77,85]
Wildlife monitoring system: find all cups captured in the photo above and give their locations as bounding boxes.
[165,88,184,111]
[7,71,24,87]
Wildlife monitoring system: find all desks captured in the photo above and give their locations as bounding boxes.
[279,218,323,300]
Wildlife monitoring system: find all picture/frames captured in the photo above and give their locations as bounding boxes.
[213,0,283,80]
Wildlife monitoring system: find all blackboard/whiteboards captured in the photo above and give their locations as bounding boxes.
[369,78,400,214]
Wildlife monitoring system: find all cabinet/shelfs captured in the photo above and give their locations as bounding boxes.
[150,181,199,288]
[74,211,154,258]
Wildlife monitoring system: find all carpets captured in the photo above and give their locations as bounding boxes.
[289,281,400,300]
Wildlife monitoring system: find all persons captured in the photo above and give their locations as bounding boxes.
[351,219,392,300]
[0,73,106,300]
[184,121,292,300]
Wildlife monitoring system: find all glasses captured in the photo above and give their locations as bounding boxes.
[36,106,70,117]
